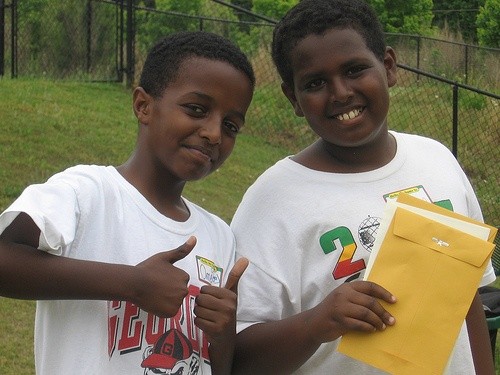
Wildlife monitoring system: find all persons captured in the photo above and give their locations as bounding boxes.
[229,0,496,375]
[0,32,256,375]
